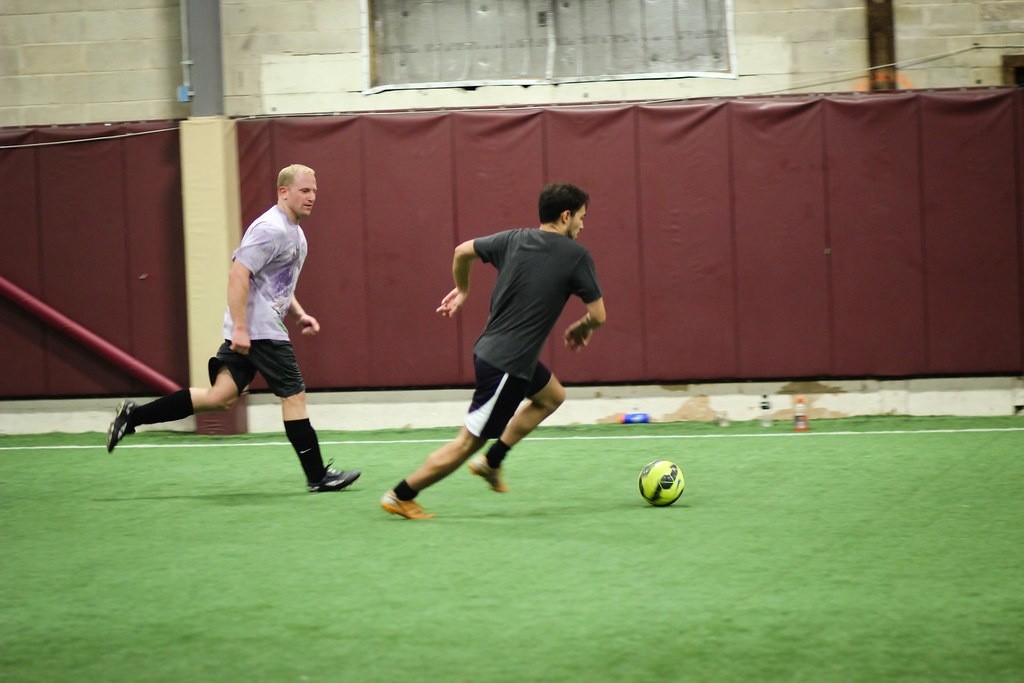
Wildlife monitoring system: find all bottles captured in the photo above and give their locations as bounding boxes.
[620,413,649,423]
[793,396,807,430]
[760,394,771,427]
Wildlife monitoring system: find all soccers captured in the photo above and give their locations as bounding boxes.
[637,459,685,507]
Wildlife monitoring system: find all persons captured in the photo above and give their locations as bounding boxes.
[105,165,362,493]
[379,182,607,519]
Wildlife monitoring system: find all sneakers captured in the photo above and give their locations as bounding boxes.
[381,490,438,519]
[107,399,135,454]
[307,457,361,493]
[468,455,507,493]
